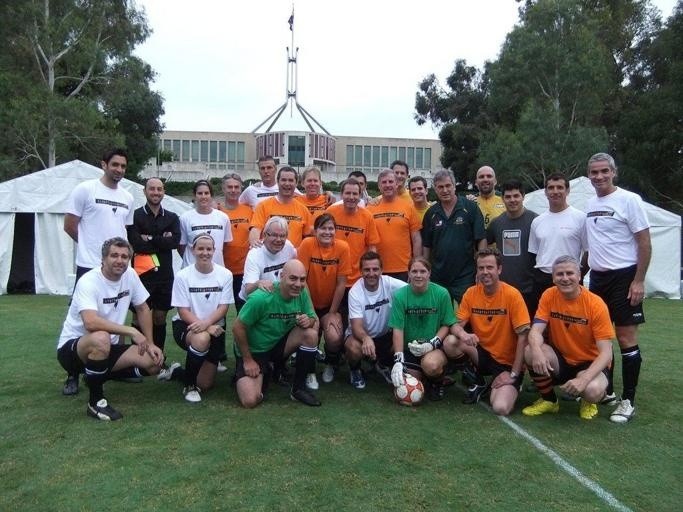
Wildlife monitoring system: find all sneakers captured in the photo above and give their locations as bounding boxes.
[288,383,321,407]
[86,398,123,422]
[182,385,202,402]
[609,396,635,423]
[520,396,560,417]
[305,373,320,390]
[463,378,488,404]
[428,384,445,401]
[63,378,79,395]
[375,360,393,384]
[321,363,335,383]
[156,361,182,381]
[575,390,616,407]
[349,368,366,389]
[578,399,599,420]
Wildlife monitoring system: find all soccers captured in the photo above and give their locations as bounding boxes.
[394,375,425,406]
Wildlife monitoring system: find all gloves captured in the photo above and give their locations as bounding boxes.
[407,335,442,357]
[390,351,405,388]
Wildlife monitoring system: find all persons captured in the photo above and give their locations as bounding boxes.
[57,148,134,396]
[153,153,539,418]
[517,254,616,421]
[57,234,165,422]
[124,176,180,372]
[527,171,588,401]
[585,148,652,422]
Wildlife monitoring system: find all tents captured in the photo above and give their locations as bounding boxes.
[0,158,193,295]
[523,176,682,301]
